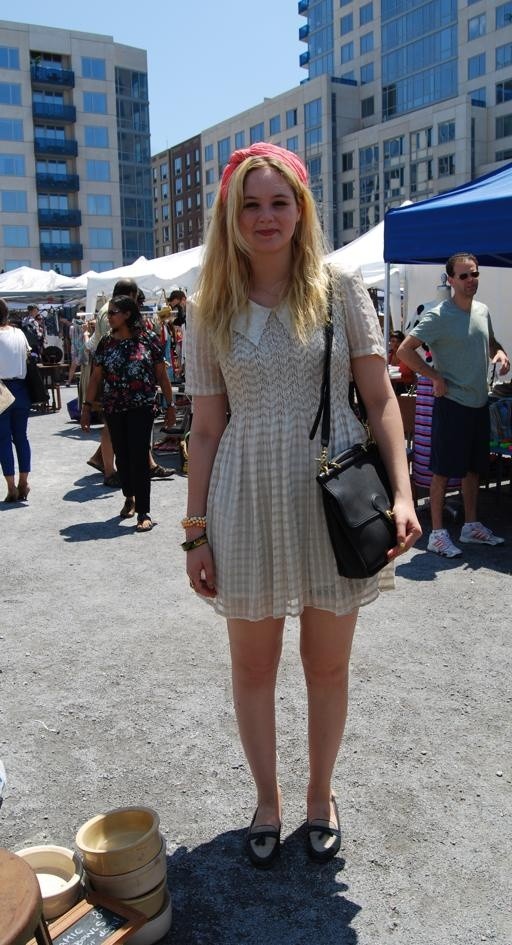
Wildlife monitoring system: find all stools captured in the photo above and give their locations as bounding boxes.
[0,846,53,945]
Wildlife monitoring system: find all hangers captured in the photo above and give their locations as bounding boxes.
[79,309,178,332]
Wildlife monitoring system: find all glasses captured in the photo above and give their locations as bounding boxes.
[107,309,124,314]
[452,271,480,280]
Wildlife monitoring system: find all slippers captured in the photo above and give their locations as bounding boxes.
[88,460,107,473]
[133,520,158,533]
[119,499,135,517]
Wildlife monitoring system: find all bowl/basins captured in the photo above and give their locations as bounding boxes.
[78,804,173,945]
[13,845,84,918]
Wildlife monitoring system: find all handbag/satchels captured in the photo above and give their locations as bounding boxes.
[23,358,51,403]
[317,442,409,577]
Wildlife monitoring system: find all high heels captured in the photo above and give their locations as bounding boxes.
[4,487,32,502]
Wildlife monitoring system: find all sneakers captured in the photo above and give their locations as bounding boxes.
[104,473,123,488]
[247,803,281,870]
[459,522,504,547]
[151,465,176,478]
[305,792,341,861]
[426,530,462,558]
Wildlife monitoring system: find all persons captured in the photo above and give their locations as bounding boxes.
[182,140,422,869]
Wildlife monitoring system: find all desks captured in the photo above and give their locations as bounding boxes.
[36,361,69,413]
